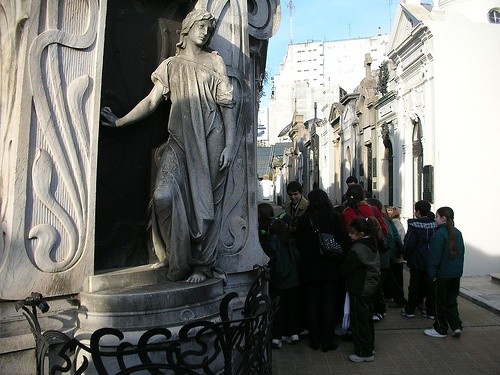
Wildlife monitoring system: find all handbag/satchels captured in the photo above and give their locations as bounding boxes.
[306,212,344,255]
[341,290,350,329]
[372,206,390,254]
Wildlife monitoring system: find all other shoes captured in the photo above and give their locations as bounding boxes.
[281,336,291,344]
[417,304,426,314]
[383,297,394,303]
[335,324,352,335]
[299,330,309,335]
[272,339,283,349]
[290,334,299,343]
[308,341,320,350]
[379,312,386,318]
[373,315,380,321]
[401,312,415,318]
[322,344,339,353]
[423,314,435,319]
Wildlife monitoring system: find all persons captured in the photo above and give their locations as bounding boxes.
[261,176,464,362]
[100,8,236,284]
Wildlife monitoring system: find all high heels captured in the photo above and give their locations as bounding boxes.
[388,303,399,308]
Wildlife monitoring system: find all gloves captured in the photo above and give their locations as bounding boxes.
[284,213,293,223]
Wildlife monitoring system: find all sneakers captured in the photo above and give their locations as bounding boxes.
[424,328,447,337]
[452,329,461,337]
[350,350,375,362]
